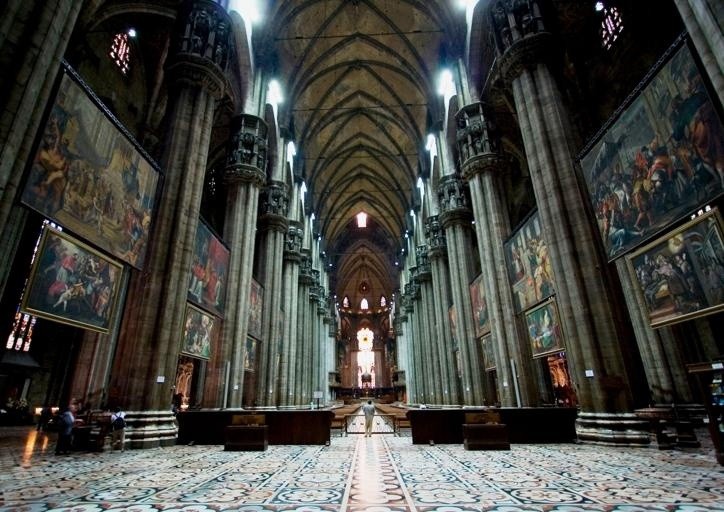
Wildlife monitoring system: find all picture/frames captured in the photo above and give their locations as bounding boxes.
[243,275,264,372]
[178,213,231,361]
[19,224,124,334]
[571,35,724,330]
[14,59,166,274]
[503,206,566,361]
[468,270,498,372]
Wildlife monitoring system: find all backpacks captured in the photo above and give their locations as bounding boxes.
[111,412,126,431]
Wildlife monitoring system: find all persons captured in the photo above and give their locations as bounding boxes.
[36,406,52,431]
[586,43,724,314]
[509,236,560,352]
[362,399,379,436]
[183,251,224,359]
[58,402,84,451]
[24,113,150,326]
[110,406,127,453]
[56,405,75,456]
